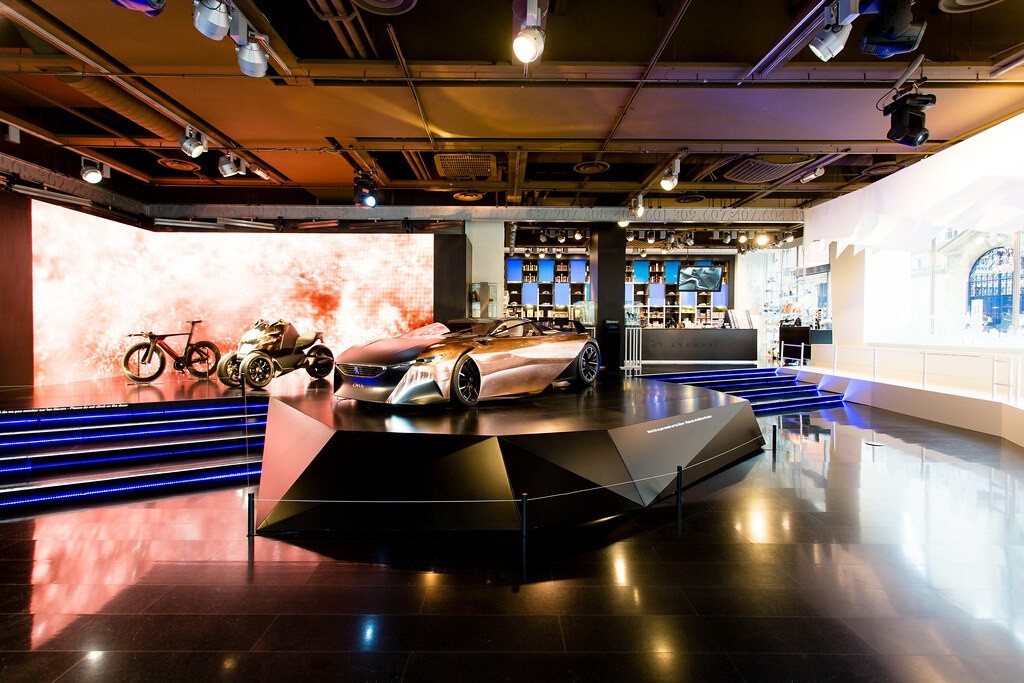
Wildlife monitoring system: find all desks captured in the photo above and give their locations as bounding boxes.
[779,326,832,364]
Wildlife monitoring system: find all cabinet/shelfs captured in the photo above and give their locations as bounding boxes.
[504,257,732,330]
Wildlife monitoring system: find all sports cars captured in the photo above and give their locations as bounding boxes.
[334,316,600,411]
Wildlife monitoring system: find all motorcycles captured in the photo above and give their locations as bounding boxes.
[217,318,335,390]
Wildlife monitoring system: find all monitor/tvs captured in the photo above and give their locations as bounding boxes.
[677,265,723,291]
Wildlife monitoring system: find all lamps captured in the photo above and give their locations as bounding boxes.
[524,221,794,259]
[513,0,544,63]
[218,150,237,177]
[353,170,378,209]
[635,194,644,217]
[80,156,102,183]
[660,158,681,191]
[800,167,825,184]
[235,31,270,77]
[809,0,937,148]
[192,0,232,41]
[178,125,204,158]
[249,164,270,180]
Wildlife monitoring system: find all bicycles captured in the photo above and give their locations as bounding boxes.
[121,319,222,383]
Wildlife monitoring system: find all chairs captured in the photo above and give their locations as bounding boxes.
[507,323,523,337]
